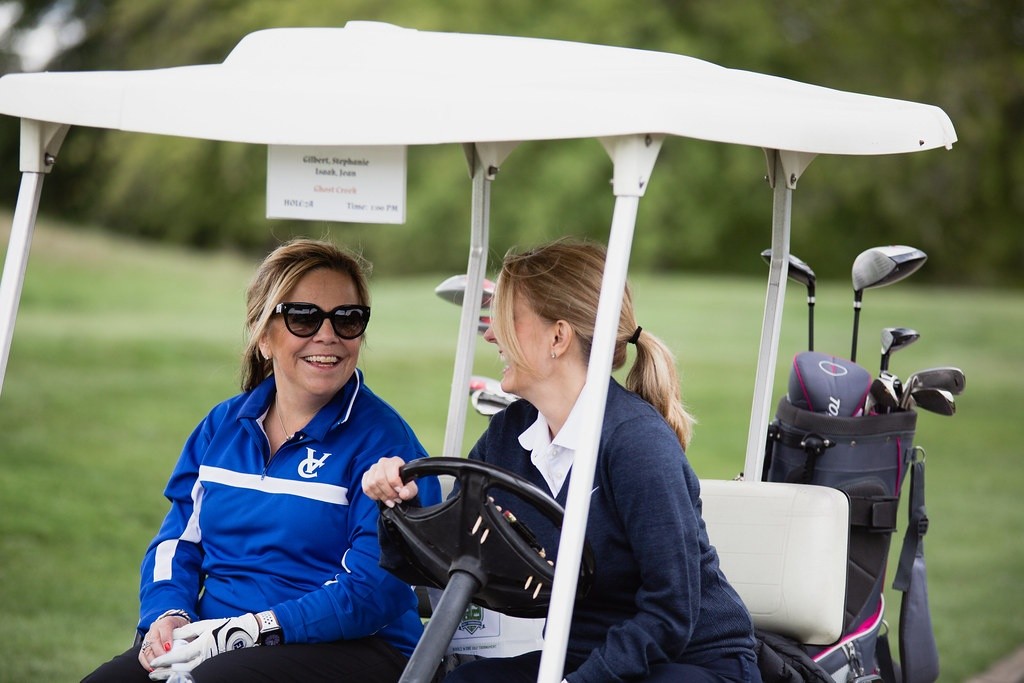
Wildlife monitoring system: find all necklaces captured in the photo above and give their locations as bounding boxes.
[275,393,292,440]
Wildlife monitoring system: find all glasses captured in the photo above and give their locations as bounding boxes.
[270,301,371,339]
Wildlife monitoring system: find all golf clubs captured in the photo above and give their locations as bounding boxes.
[865,366,969,416]
[880,323,921,371]
[435,273,534,404]
[762,246,818,352]
[850,245,929,362]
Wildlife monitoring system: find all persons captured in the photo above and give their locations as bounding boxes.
[79,238,443,683]
[362,242,763,683]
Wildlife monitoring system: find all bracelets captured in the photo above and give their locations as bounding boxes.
[155,609,191,622]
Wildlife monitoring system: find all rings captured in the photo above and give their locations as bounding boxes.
[142,642,149,652]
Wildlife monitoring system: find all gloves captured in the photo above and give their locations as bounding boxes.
[148,613,259,681]
